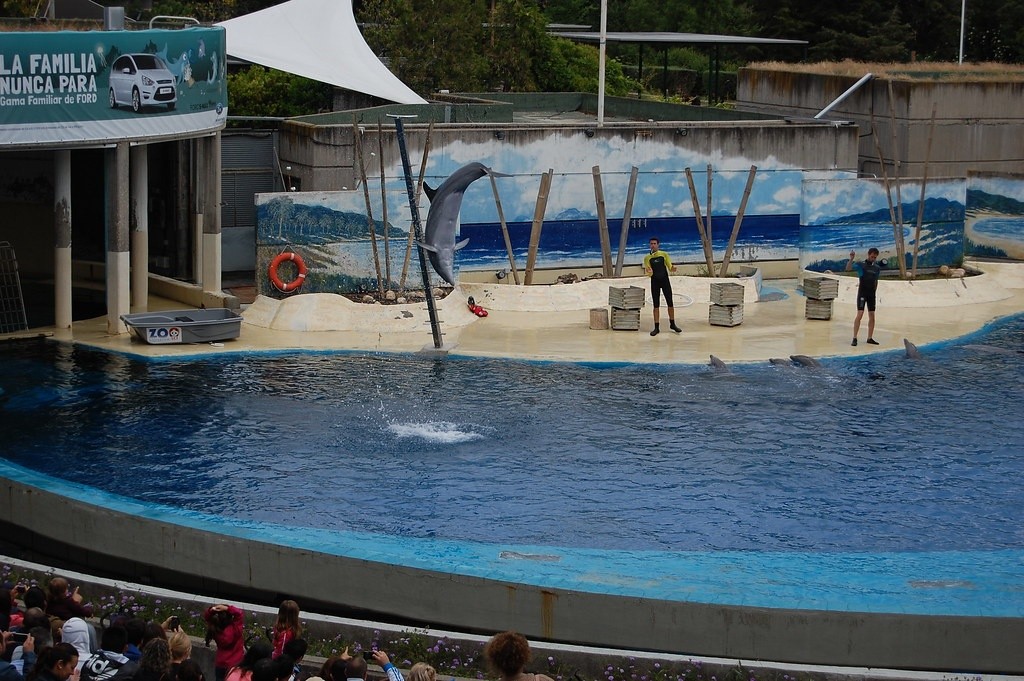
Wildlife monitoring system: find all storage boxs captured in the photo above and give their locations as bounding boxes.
[803,277,839,320]
[609,286,645,331]
[709,283,744,327]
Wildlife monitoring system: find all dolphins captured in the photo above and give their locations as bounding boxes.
[412,159,515,287]
[706,337,1024,377]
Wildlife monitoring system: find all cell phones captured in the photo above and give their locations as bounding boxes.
[17,586,25,593]
[13,633,28,642]
[363,652,376,661]
[171,618,180,632]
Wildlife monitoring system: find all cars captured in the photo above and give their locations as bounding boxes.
[109,53,179,113]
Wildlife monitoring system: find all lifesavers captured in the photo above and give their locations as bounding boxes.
[268,252,307,291]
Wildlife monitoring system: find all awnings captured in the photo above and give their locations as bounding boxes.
[212,0,430,104]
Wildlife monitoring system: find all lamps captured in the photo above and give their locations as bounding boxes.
[585,128,594,137]
[675,128,688,136]
[496,271,506,279]
[497,132,506,140]
[879,258,888,266]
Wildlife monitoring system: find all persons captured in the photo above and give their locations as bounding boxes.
[643,238,683,336]
[0,566,555,681]
[846,247,881,346]
